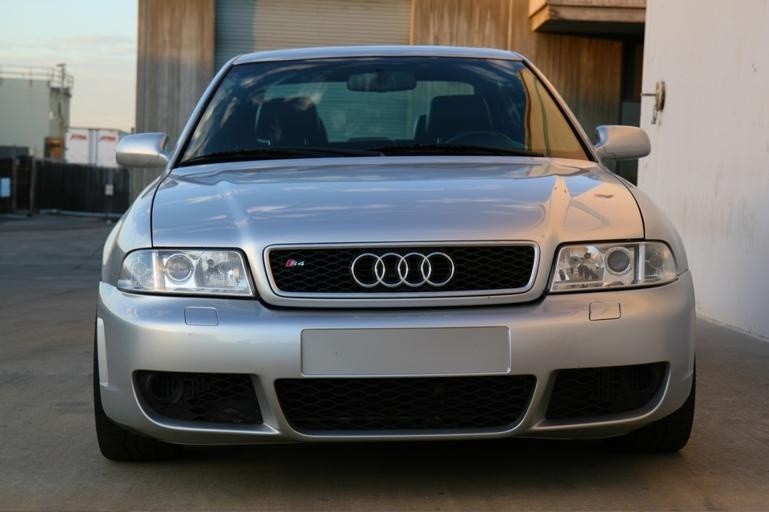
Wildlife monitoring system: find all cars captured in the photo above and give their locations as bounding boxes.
[92,46,697,461]
